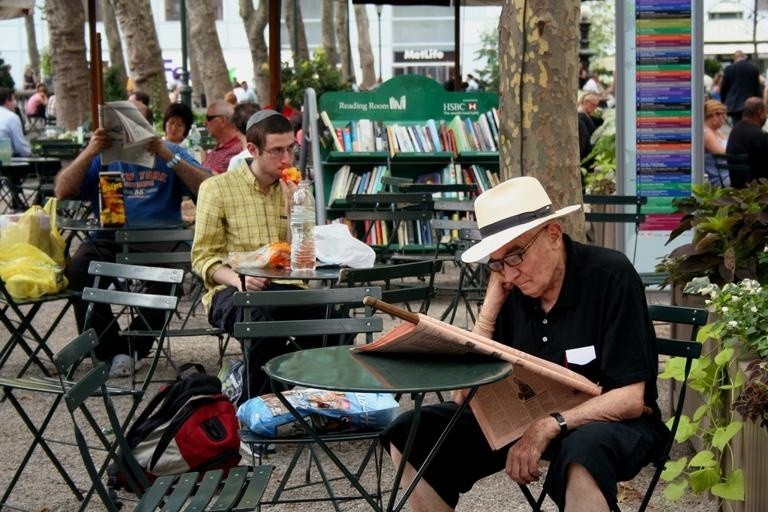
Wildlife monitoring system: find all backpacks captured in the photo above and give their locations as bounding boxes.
[104,362,242,511]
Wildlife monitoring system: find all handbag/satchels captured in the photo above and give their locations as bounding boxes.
[236,388,402,437]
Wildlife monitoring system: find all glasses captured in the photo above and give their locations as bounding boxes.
[486,223,550,272]
[206,114,229,121]
[258,139,301,159]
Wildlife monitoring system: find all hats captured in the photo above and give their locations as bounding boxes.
[461,175,581,264]
[245,108,280,131]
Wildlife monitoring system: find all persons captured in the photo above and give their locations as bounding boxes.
[128,92,149,107]
[0,59,59,210]
[443,78,470,92]
[160,103,201,162]
[191,110,314,456]
[54,104,214,378]
[204,78,261,174]
[702,50,767,190]
[579,67,616,177]
[378,177,664,511]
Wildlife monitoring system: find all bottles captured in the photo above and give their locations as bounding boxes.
[289,180,316,272]
[45,115,57,140]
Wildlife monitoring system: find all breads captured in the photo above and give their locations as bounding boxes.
[270,243,291,267]
[282,166,303,186]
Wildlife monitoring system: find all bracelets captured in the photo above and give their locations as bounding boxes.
[476,312,495,335]
[167,154,181,167]
[549,412,568,435]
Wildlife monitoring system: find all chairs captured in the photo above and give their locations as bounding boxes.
[0,140,709,512]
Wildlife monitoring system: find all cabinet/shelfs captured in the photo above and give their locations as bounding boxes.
[298,73,501,248]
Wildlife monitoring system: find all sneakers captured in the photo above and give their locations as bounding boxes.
[108,354,133,379]
[216,358,243,407]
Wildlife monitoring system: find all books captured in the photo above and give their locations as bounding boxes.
[321,107,500,247]
